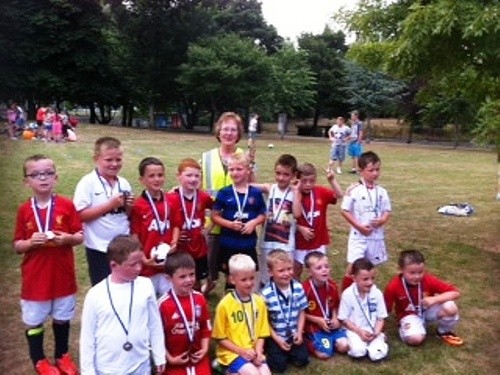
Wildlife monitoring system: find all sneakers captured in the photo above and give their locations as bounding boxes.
[54,354,79,375]
[351,168,356,173]
[326,168,332,173]
[336,166,342,173]
[33,358,60,375]
[435,328,463,346]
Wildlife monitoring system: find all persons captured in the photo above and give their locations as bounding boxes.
[338,258,389,361]
[156,249,214,375]
[166,110,259,301]
[379,250,464,347]
[345,108,368,174]
[301,251,350,359]
[292,162,342,282]
[262,247,310,373]
[2,101,80,145]
[73,136,137,290]
[337,150,392,269]
[326,116,352,173]
[244,111,259,152]
[211,252,273,375]
[126,157,184,273]
[78,234,167,375]
[168,157,217,295]
[208,153,268,276]
[12,153,85,375]
[247,152,301,287]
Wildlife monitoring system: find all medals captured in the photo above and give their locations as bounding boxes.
[123,342,132,351]
[44,231,55,240]
